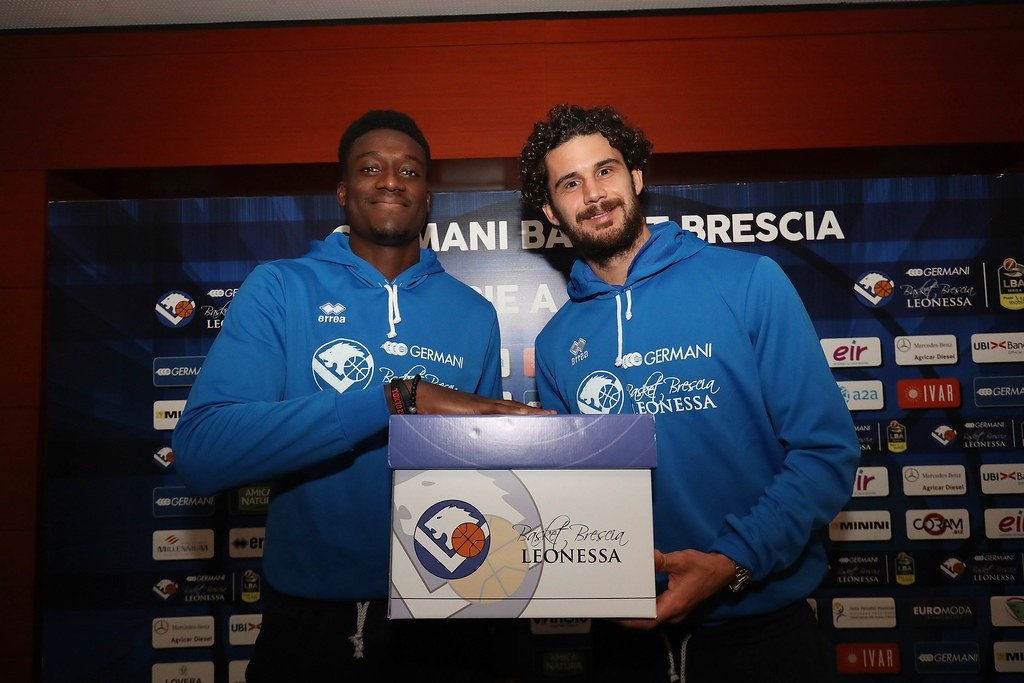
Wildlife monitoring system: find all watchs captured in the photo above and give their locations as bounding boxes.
[391,378,413,414]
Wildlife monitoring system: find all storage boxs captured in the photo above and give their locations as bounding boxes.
[386,412,658,619]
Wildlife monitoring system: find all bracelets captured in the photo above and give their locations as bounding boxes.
[729,559,751,593]
[409,374,421,415]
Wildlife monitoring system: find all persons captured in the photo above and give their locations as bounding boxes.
[519,102,862,683]
[171,109,557,683]
